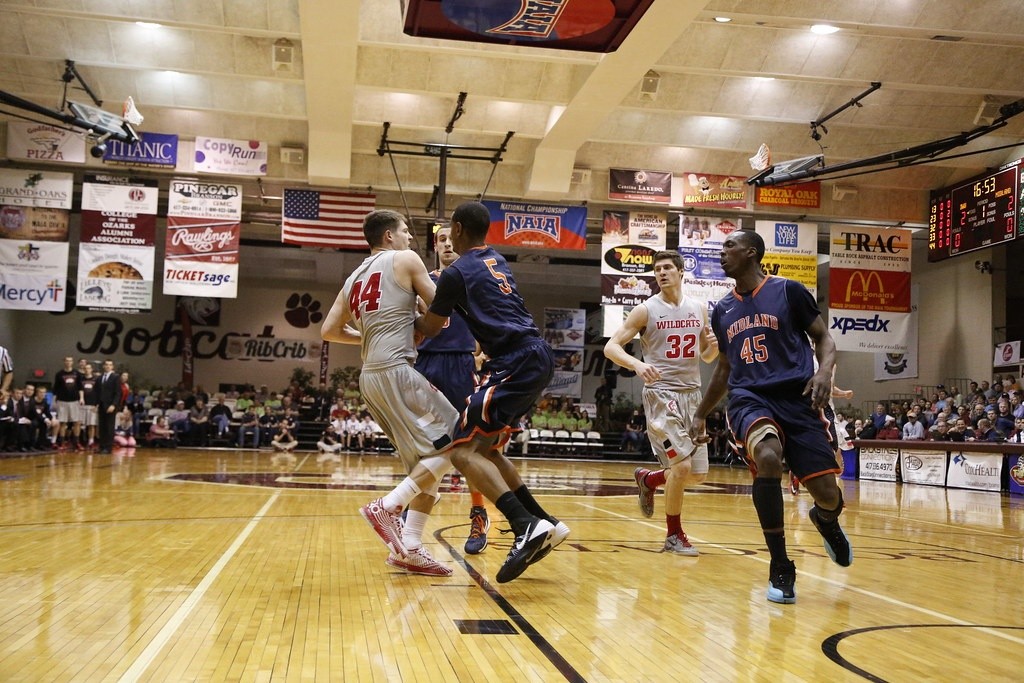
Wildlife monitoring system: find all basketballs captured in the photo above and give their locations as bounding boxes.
[415,296,427,346]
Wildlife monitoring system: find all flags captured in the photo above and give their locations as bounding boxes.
[480,199,588,250]
[827,223,918,382]
[281,187,376,249]
[994,341,1021,367]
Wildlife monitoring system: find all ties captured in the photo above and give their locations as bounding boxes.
[104,374,107,384]
[16,402,19,419]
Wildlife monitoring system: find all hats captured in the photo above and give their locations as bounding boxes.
[936,385,945,389]
[908,413,917,418]
[1001,393,1009,399]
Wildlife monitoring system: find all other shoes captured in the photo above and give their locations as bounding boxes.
[6,440,97,454]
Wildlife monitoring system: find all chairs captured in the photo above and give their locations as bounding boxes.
[507,426,605,460]
[130,377,402,454]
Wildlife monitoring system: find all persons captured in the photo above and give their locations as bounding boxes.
[603,250,719,557]
[689,229,852,604]
[706,405,735,459]
[0,346,313,454]
[836,374,1024,442]
[501,393,593,456]
[315,380,376,454]
[618,410,652,452]
[414,201,571,583]
[788,338,856,496]
[401,222,491,554]
[595,378,613,433]
[320,209,461,577]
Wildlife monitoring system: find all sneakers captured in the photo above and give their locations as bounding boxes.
[464,509,491,554]
[635,466,655,518]
[809,507,852,567]
[664,534,699,556]
[496,517,555,582]
[767,558,796,604]
[530,515,571,564]
[360,496,410,561]
[789,471,799,496]
[383,544,453,577]
